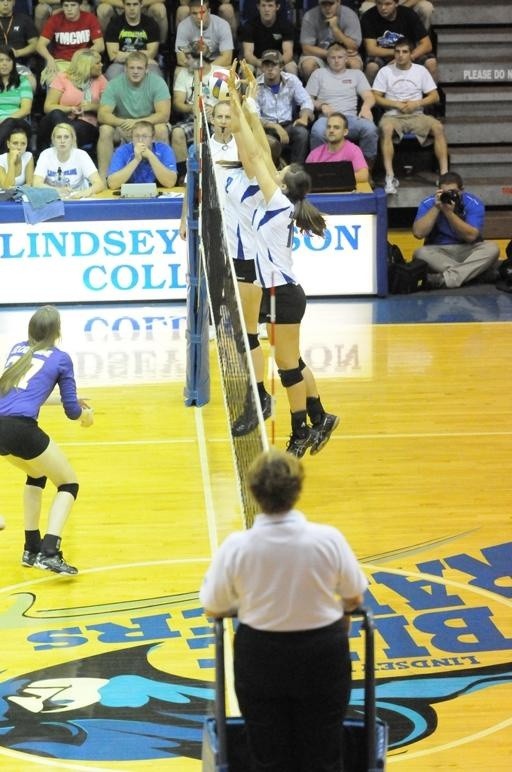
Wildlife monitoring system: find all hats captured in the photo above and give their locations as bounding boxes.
[260,48,283,65]
[177,36,220,60]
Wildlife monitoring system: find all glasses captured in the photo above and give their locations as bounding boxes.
[130,135,154,141]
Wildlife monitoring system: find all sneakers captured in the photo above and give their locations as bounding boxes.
[21,548,80,577]
[308,412,341,458]
[230,391,277,439]
[424,270,447,293]
[282,428,321,463]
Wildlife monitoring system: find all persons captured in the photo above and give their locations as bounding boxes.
[181,54,339,460]
[201,449,367,771]
[0,0,498,289]
[2,306,94,576]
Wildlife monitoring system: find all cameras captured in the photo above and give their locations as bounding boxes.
[440,189,461,204]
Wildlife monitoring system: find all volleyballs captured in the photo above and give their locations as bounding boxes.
[207,68,241,101]
